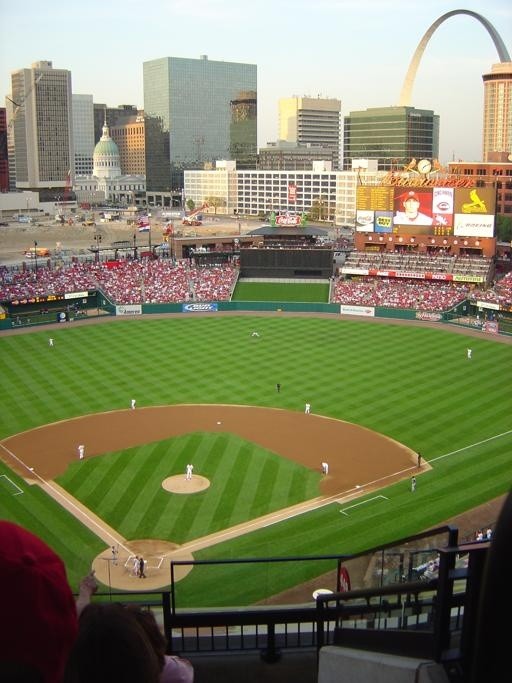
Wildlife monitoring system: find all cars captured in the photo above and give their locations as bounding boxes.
[0,209,205,233]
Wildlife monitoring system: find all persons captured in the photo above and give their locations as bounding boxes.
[48,337,55,349]
[487,527,493,539]
[411,476,417,491]
[320,461,328,475]
[304,401,311,415]
[130,399,135,413]
[476,528,485,540]
[139,557,146,578]
[76,569,98,617]
[1,243,242,327]
[0,518,76,682]
[185,462,194,478]
[77,440,85,459]
[466,347,473,358]
[244,236,330,249]
[417,451,422,467]
[65,601,161,682]
[126,603,194,682]
[133,555,140,575]
[276,382,281,392]
[111,544,120,565]
[331,241,512,336]
[394,190,434,225]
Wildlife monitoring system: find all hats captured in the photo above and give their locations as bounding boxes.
[0,519,80,683]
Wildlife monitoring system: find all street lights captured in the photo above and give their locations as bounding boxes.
[92,232,103,261]
[33,239,39,272]
[132,232,137,261]
